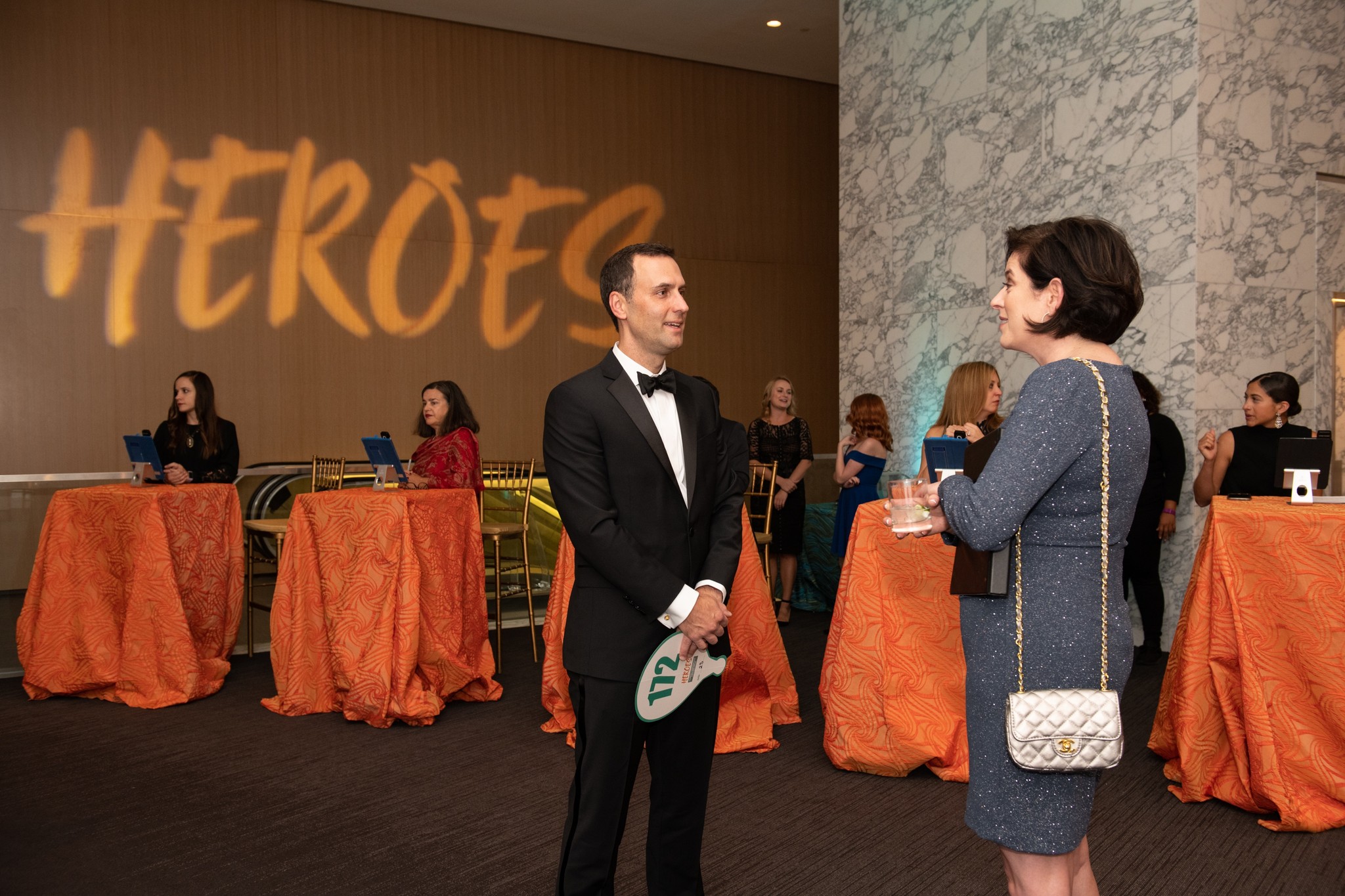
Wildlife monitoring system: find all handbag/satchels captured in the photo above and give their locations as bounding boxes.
[1003,689,1124,772]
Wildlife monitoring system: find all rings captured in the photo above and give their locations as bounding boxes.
[968,431,970,436]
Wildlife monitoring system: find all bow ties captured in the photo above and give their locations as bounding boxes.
[636,369,677,398]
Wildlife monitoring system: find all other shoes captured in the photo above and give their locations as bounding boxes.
[1132,638,1163,666]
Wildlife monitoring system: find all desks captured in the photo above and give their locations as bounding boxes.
[537,500,800,753]
[819,496,970,786]
[265,483,499,726]
[19,482,245,713]
[1148,490,1345,835]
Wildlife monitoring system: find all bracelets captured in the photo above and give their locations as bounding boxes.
[1162,508,1177,516]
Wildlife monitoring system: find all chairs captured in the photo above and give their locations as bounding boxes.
[473,458,538,674]
[244,455,348,664]
[742,461,779,605]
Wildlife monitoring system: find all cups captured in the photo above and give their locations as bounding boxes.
[888,478,932,533]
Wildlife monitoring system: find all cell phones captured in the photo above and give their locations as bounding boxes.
[1227,492,1253,500]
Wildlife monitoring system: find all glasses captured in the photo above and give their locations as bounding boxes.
[401,482,429,490]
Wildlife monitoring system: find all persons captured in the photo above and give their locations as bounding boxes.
[825,394,894,634]
[1192,372,1325,506]
[133,371,240,485]
[1121,370,1187,664]
[544,242,745,896]
[918,361,1007,485]
[884,218,1152,896]
[748,376,814,627]
[395,380,485,493]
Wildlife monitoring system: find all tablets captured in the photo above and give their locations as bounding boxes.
[360,431,408,483]
[922,430,969,484]
[1276,429,1333,490]
[123,430,164,480]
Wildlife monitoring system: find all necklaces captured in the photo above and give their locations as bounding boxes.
[432,435,436,440]
[183,428,201,448]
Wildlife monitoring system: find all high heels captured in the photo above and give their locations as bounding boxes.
[776,598,792,627]
